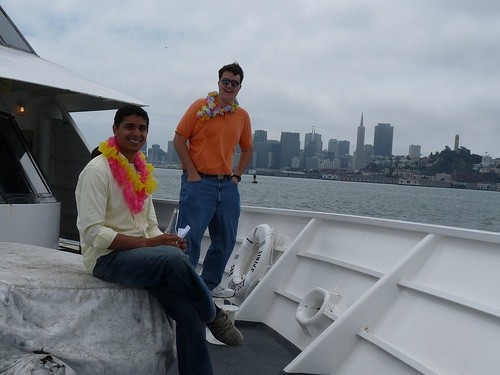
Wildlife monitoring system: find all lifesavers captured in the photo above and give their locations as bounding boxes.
[228,222,275,298]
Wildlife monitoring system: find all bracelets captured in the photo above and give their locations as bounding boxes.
[232,173,242,182]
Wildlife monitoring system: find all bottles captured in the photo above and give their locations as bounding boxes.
[163,208,179,244]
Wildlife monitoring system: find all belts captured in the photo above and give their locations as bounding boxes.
[197,172,232,181]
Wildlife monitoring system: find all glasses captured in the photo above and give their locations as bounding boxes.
[221,78,240,87]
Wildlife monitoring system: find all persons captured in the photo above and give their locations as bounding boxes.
[170,60,254,297]
[253,172,257,181]
[77,104,244,375]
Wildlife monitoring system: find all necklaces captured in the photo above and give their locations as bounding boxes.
[98,135,158,215]
[194,91,241,122]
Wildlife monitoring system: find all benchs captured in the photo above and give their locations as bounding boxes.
[0,243,177,375]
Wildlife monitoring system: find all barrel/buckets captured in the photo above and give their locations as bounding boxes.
[205,304,239,345]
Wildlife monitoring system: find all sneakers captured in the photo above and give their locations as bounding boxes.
[207,307,244,347]
[209,285,235,298]
